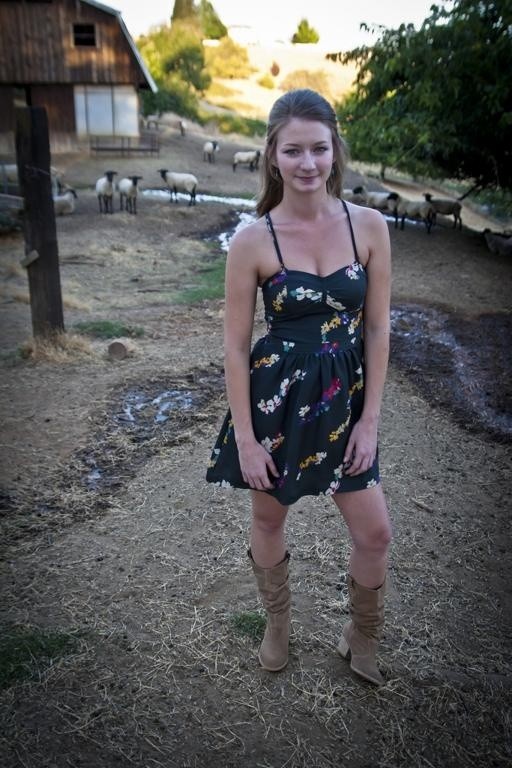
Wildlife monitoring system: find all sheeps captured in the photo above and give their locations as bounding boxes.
[52,188,79,216]
[480,228,512,260]
[232,149,262,172]
[203,140,219,163]
[95,169,143,214]
[342,185,463,235]
[156,169,198,206]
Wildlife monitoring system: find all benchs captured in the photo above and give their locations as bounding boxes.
[90,134,160,158]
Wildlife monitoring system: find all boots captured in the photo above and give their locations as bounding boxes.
[337,572,385,686]
[248,549,291,672]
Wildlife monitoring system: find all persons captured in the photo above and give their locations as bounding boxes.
[205,89,391,686]
